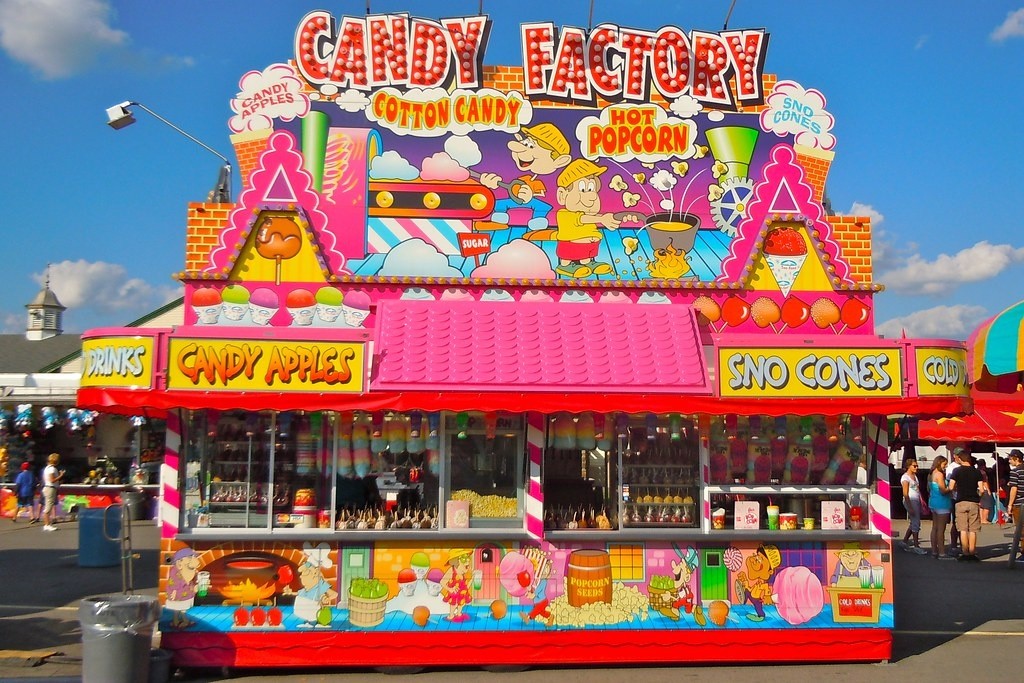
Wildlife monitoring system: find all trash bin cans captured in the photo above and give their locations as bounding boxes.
[119,489,148,521]
[77,593,160,683]
[78,507,123,569]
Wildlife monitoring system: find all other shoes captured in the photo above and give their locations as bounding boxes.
[27,518,40,525]
[10,517,18,522]
[981,521,993,525]
[52,518,56,524]
[960,553,980,565]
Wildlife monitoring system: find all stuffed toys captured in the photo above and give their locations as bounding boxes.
[0,404,148,485]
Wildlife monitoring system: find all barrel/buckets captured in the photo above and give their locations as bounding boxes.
[293,506,317,528]
[318,510,337,528]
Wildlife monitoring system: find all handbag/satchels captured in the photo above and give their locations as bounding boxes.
[919,496,932,520]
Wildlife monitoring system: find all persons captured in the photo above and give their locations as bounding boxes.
[35,460,59,523]
[11,461,39,525]
[889,446,1024,563]
[42,452,67,532]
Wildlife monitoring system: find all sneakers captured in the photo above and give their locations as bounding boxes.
[913,546,928,556]
[931,552,957,562]
[898,540,910,553]
[1015,555,1024,563]
[948,548,963,556]
[44,525,59,532]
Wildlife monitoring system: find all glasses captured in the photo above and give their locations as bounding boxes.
[912,465,920,468]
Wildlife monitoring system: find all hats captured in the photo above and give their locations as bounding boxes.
[952,446,967,456]
[20,462,31,470]
[1006,449,1024,459]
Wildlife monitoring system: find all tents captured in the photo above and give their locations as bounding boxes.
[889,364,1024,524]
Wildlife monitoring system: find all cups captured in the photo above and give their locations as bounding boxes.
[803,518,815,530]
[711,508,725,529]
[779,513,797,530]
[766,506,779,530]
